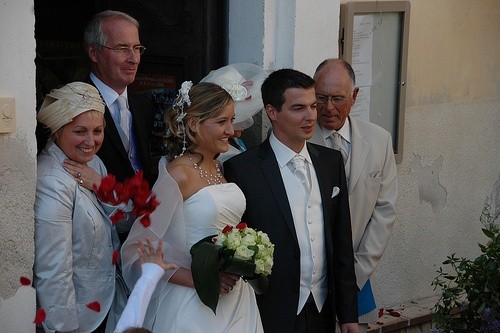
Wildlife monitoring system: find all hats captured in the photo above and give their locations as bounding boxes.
[37,81,105,133]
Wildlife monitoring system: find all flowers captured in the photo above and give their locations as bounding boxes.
[212,222,275,277]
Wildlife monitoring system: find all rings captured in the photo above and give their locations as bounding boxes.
[80,179,82,185]
[77,172,81,178]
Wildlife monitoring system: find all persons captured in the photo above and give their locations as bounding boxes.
[112,236,176,333]
[306,58,399,321]
[222,68,359,333]
[32,81,132,333]
[70,9,169,245]
[103,63,271,333]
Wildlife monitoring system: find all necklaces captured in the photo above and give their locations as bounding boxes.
[183,150,223,186]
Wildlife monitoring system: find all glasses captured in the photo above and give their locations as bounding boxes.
[316,90,353,106]
[99,45,147,56]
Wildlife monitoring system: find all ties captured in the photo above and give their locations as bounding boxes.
[291,156,311,195]
[330,132,348,167]
